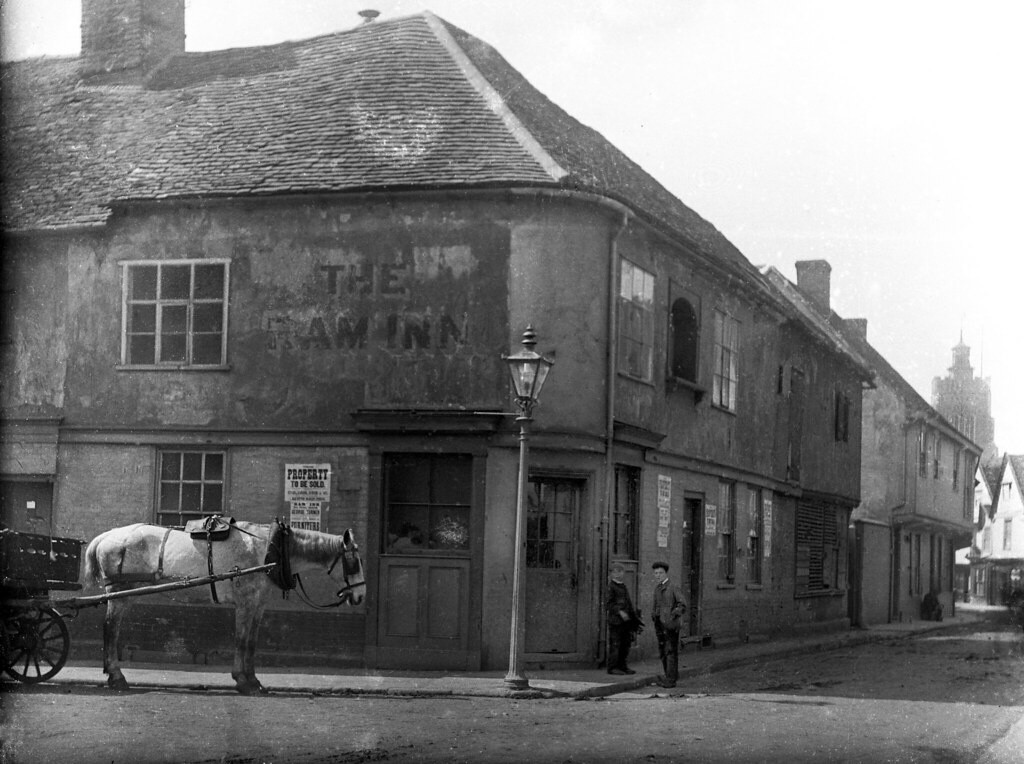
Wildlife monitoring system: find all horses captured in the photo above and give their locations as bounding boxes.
[83,521,367,696]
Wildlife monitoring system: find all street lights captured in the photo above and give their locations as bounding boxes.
[491,323,558,693]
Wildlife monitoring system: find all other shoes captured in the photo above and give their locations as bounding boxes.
[618,665,635,674]
[608,669,626,675]
[657,678,676,688]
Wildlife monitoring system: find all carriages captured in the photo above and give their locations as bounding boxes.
[2,516,370,692]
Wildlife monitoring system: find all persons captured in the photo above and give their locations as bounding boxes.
[651,562,688,688]
[607,563,643,676]
[923,588,945,622]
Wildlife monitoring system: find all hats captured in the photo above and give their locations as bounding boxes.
[652,561,669,573]
